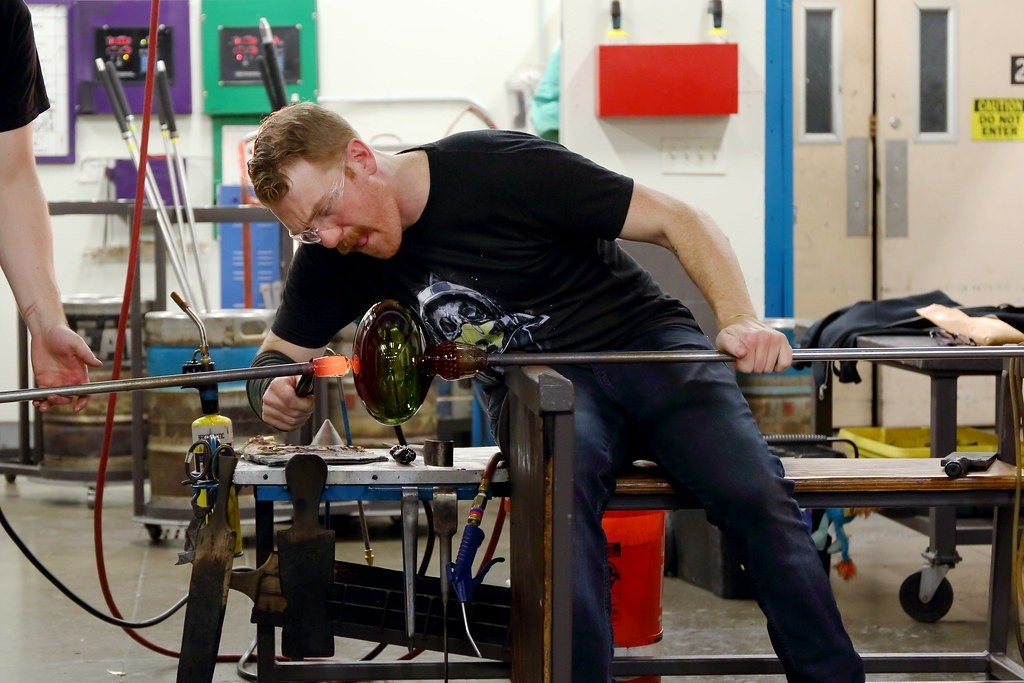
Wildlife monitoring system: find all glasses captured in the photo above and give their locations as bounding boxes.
[930,328,978,349]
[290,147,347,244]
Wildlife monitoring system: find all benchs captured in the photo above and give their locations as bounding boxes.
[605,456,1024,511]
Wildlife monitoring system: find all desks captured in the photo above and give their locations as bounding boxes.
[792,322,1024,624]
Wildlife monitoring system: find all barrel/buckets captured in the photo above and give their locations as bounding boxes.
[597,511,666,648]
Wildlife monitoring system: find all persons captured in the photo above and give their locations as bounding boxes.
[244,103,865,683]
[0,0,102,413]
